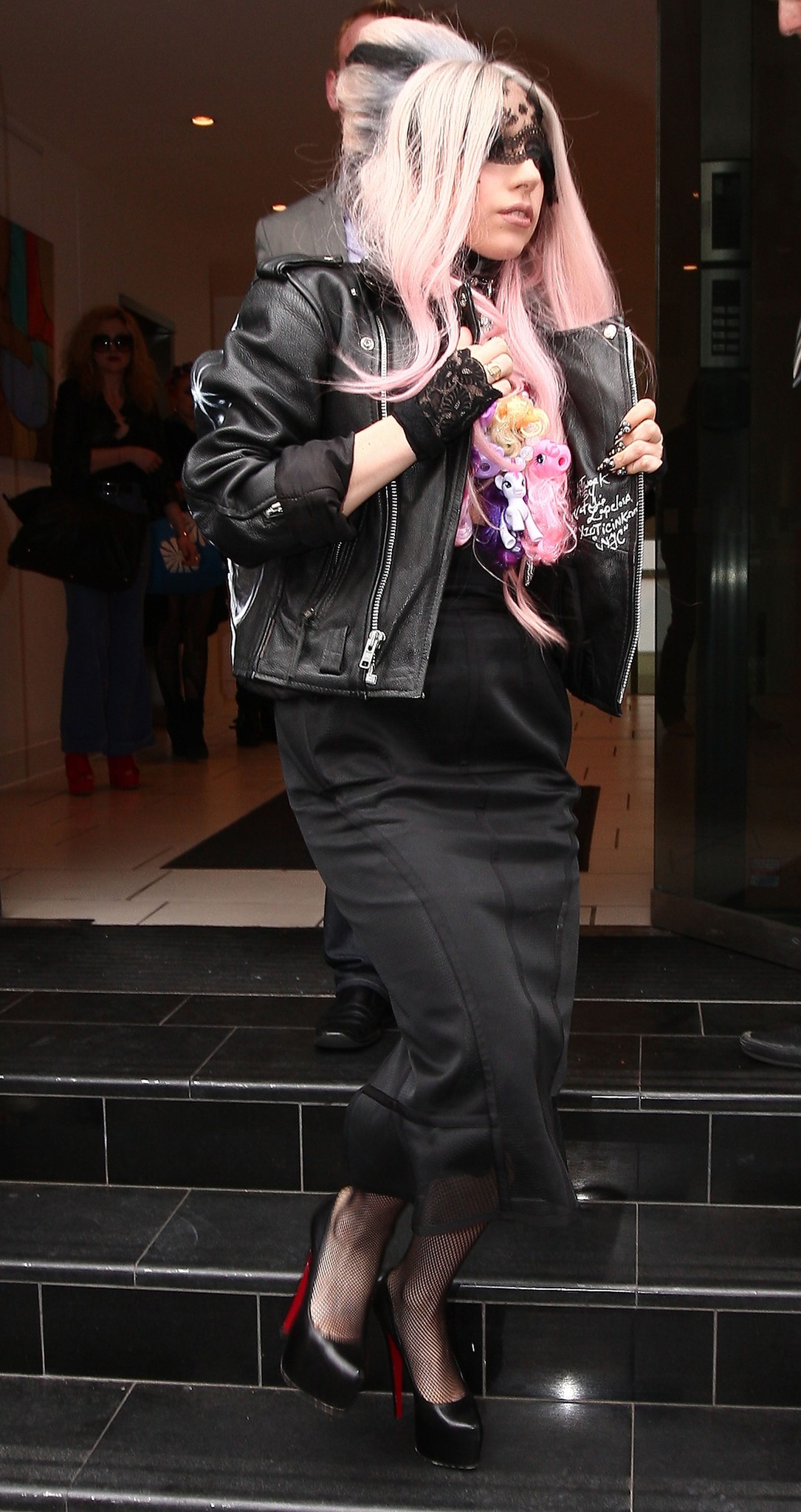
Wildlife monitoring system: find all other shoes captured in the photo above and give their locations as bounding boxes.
[105,753,146,790]
[65,750,95,795]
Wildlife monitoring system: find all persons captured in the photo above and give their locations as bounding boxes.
[179,8,663,1473]
[37,301,229,796]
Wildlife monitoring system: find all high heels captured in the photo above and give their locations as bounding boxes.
[279,1194,367,1418]
[373,1268,482,1470]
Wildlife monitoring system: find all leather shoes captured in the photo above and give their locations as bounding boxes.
[315,983,386,1051]
[740,1023,801,1067]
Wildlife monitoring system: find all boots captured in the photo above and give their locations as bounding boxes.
[164,695,209,763]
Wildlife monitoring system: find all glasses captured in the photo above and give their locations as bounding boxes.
[90,332,135,353]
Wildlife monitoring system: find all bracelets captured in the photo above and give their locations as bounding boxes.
[116,442,124,460]
